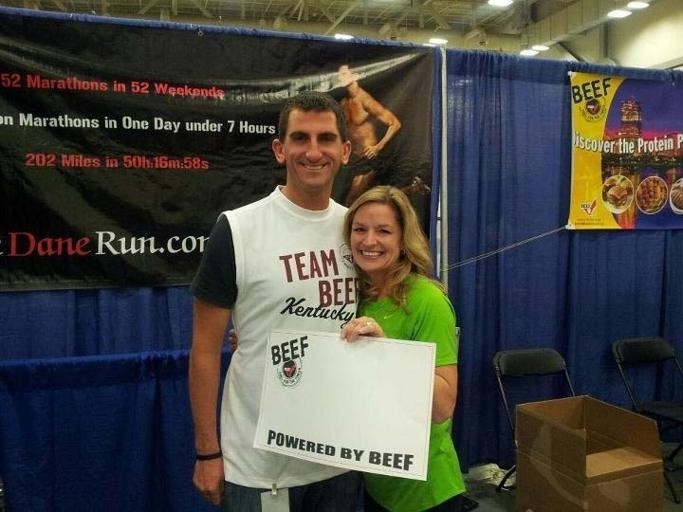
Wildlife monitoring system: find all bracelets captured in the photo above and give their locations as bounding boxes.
[194,451,223,463]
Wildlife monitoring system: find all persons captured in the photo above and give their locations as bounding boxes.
[340,185,476,512]
[334,61,431,208]
[185,88,362,510]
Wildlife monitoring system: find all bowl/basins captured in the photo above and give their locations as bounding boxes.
[600,175,683,216]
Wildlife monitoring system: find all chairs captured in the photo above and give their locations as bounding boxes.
[496,338,683,510]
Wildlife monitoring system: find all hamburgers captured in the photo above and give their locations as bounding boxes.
[607,185,628,206]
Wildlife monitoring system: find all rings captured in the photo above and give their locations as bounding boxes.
[350,324,354,329]
[367,322,372,326]
